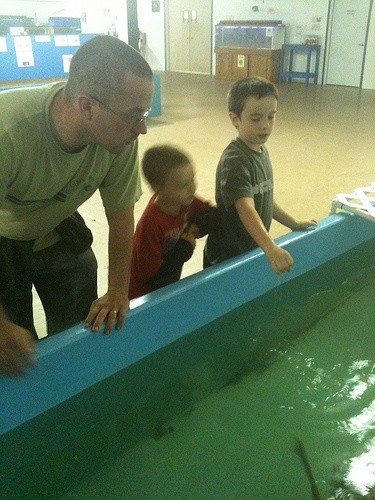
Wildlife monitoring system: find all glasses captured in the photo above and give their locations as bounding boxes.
[84,94,155,127]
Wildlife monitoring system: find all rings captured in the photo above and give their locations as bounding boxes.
[110,309,118,315]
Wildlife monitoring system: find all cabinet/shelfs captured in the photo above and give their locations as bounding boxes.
[212,48,284,86]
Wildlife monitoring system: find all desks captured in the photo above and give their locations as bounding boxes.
[278,44,320,87]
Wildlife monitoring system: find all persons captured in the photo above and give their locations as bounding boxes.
[0,34,156,381]
[138,28,146,56]
[201,75,318,275]
[127,146,219,302]
[108,23,118,39]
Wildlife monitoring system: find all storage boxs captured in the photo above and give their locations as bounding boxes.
[215,20,287,48]
